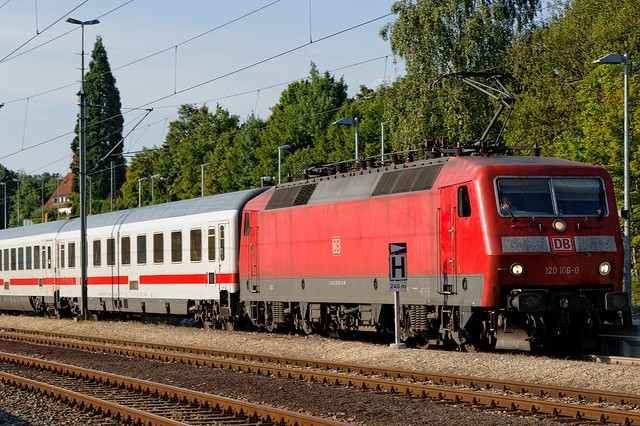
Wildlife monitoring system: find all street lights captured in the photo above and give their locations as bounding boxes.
[200,163,212,197]
[85,174,93,215]
[67,18,100,316]
[1,181,8,232]
[151,173,159,203]
[331,116,360,161]
[37,177,45,222]
[276,144,292,185]
[57,176,65,219]
[138,177,148,207]
[591,52,632,324]
[12,177,21,226]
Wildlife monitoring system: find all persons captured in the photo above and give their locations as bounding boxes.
[500,198,516,210]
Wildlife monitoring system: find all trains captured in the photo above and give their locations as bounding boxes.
[0,70,629,359]
